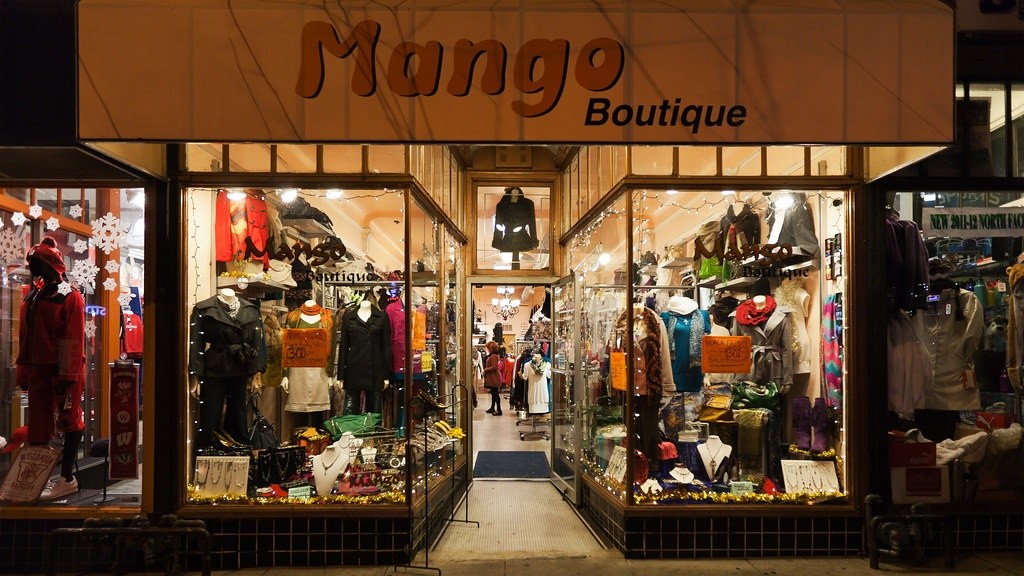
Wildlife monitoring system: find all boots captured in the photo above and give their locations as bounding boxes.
[791,396,829,453]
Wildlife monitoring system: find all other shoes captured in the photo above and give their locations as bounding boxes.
[485,408,495,413]
[492,411,502,416]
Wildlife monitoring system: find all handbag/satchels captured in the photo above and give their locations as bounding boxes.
[249,390,279,449]
[698,378,781,424]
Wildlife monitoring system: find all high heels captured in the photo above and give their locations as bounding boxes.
[210,427,244,451]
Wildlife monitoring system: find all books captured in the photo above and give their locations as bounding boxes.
[676,428,700,443]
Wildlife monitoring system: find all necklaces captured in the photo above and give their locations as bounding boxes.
[784,460,840,496]
[608,448,627,477]
[192,432,376,502]
[672,469,690,479]
[704,441,723,478]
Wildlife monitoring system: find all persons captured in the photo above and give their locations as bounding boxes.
[609,192,828,465]
[186,189,425,452]
[503,353,555,415]
[482,341,502,417]
[481,344,508,394]
[491,186,539,253]
[11,234,87,498]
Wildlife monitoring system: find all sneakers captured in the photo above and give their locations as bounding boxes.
[40,476,79,501]
[0,435,7,448]
[986,401,1006,415]
[906,427,988,467]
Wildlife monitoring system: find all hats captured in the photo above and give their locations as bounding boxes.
[2,426,29,453]
[26,236,66,277]
[486,341,499,353]
